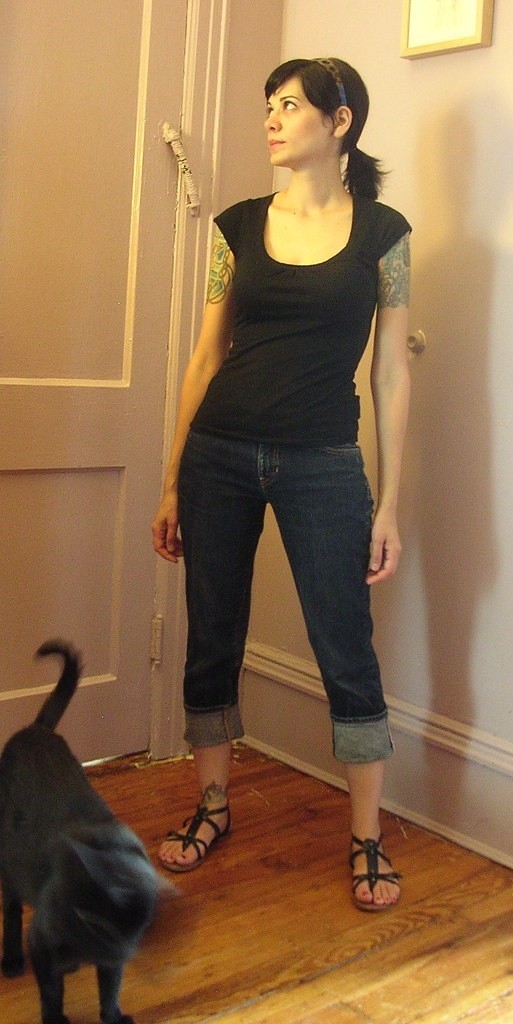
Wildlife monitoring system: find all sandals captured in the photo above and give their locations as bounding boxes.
[348,831,401,912]
[159,801,233,872]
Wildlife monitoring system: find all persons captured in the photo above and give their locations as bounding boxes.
[144,58,411,911]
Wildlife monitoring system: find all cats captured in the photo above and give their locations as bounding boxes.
[0,636,180,1024]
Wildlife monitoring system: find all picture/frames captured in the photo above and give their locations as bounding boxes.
[398,0,496,62]
